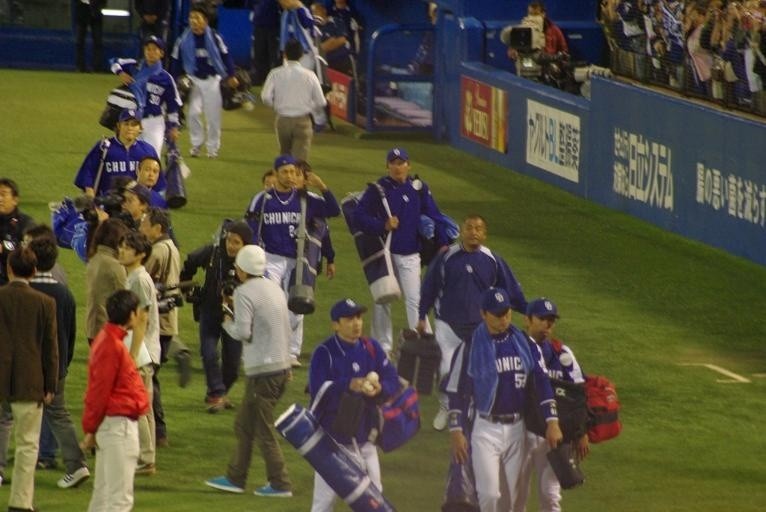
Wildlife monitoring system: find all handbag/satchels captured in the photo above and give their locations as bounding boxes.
[221,65,253,110]
[585,373,623,445]
[546,444,584,490]
[374,377,420,454]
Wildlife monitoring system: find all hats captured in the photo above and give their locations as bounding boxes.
[387,149,410,163]
[526,297,560,320]
[477,287,513,313]
[226,222,266,276]
[275,154,299,171]
[118,35,164,205]
[330,299,368,320]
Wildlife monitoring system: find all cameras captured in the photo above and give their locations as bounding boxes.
[224,279,239,296]
[83,193,126,220]
[156,280,197,314]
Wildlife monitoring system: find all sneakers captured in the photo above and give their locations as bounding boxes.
[433,405,451,431]
[37,351,294,498]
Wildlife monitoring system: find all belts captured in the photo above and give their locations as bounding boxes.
[490,414,522,424]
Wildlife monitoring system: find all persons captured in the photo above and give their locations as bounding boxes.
[303,298,401,511]
[418,212,531,430]
[1,1,355,512]
[515,297,589,511]
[440,289,564,511]
[353,145,450,363]
[509,0,765,120]
